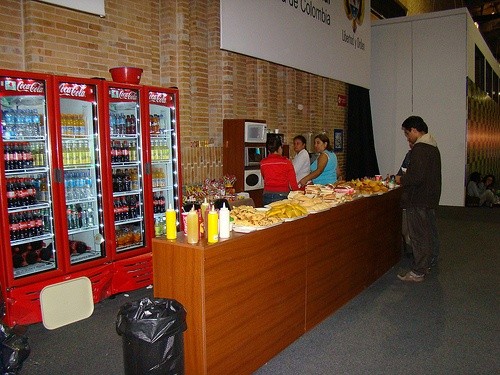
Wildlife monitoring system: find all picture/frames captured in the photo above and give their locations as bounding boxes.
[334,129,343,152]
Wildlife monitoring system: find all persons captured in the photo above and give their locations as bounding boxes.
[397,143,412,247]
[467,171,497,206]
[259,138,299,207]
[298,134,340,187]
[395,116,442,281]
[289,135,313,194]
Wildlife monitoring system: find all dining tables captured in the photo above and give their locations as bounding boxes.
[152,182,401,375]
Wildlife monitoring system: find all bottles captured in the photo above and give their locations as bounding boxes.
[108,111,140,247]
[219,202,230,239]
[205,206,210,239]
[165,203,177,240]
[149,113,171,237]
[60,112,93,257]
[2,108,54,268]
[201,196,210,220]
[207,205,219,243]
[186,208,199,244]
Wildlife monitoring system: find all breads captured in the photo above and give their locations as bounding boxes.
[230,178,390,226]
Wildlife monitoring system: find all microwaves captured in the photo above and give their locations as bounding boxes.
[244,147,267,167]
[244,122,267,143]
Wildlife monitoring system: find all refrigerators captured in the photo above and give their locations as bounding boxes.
[0,68,111,328]
[104,81,182,295]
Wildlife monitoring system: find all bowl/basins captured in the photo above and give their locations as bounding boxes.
[109,66,143,85]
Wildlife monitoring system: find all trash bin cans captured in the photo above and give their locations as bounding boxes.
[116,298,188,375]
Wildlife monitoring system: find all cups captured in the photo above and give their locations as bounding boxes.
[275,129,279,133]
[181,212,188,236]
[375,175,382,181]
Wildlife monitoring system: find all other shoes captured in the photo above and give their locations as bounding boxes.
[425,263,432,272]
[397,271,425,282]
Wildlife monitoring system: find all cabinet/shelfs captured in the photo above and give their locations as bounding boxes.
[223,119,289,192]
